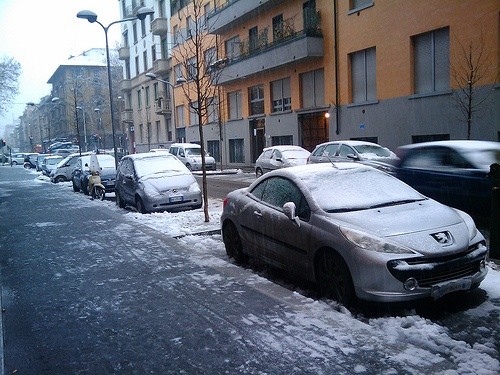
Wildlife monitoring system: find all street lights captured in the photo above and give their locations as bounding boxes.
[146,72,187,143]
[77,7,155,172]
[52,97,87,152]
[26,102,51,154]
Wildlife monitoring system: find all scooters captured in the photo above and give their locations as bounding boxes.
[84,163,106,201]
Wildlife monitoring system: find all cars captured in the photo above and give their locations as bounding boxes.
[115,152,203,214]
[0,148,120,195]
[221,162,489,313]
[255,140,500,201]
[149,142,216,172]
